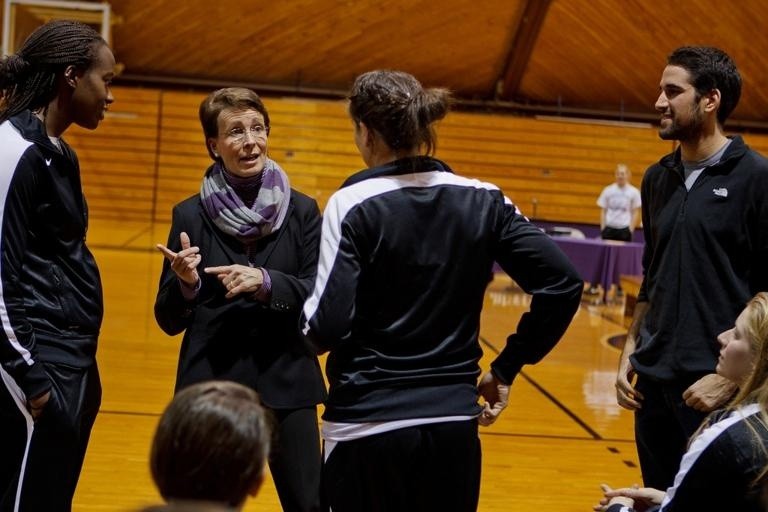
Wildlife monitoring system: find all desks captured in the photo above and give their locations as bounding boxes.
[546,233,644,303]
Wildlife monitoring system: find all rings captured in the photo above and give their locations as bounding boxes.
[229,280,235,288]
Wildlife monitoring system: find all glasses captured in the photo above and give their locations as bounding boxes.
[218,126,269,138]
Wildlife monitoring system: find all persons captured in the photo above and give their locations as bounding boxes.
[298,70,584,511]
[614,44,767,512]
[585,163,642,297]
[139,381,271,512]
[154,89,327,512]
[594,292,767,512]
[0,17,115,512]
